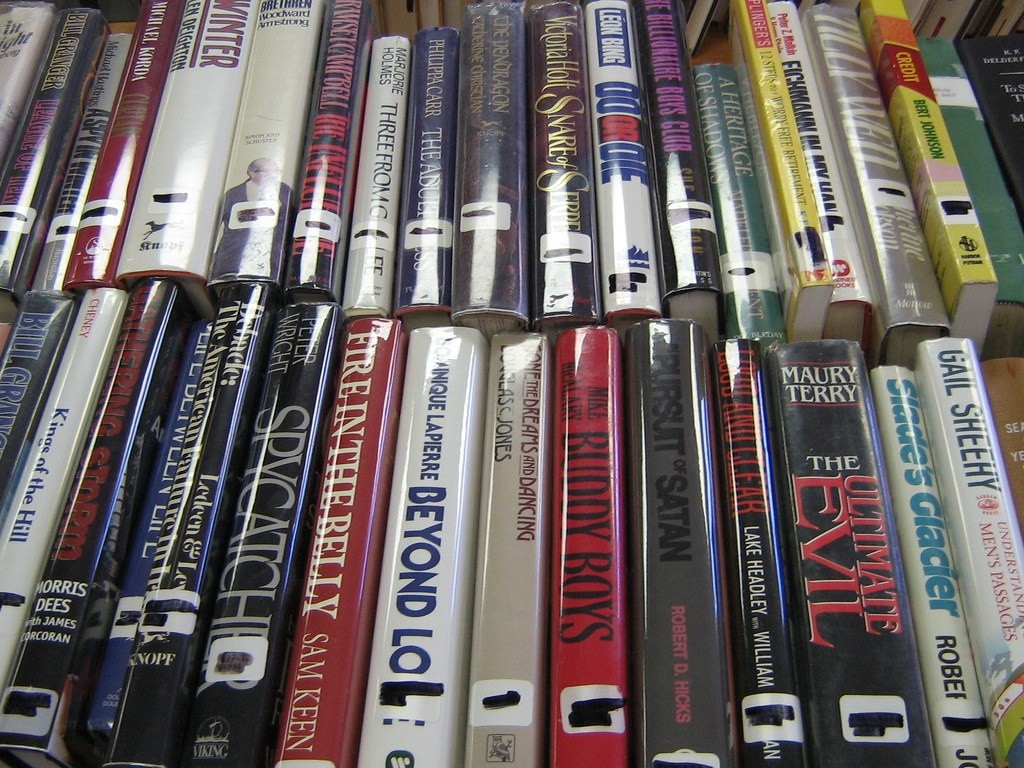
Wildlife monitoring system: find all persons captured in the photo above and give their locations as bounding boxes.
[211,158,294,280]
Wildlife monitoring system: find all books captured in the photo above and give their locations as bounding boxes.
[0,277,1024,768]
[0,0,1024,368]
